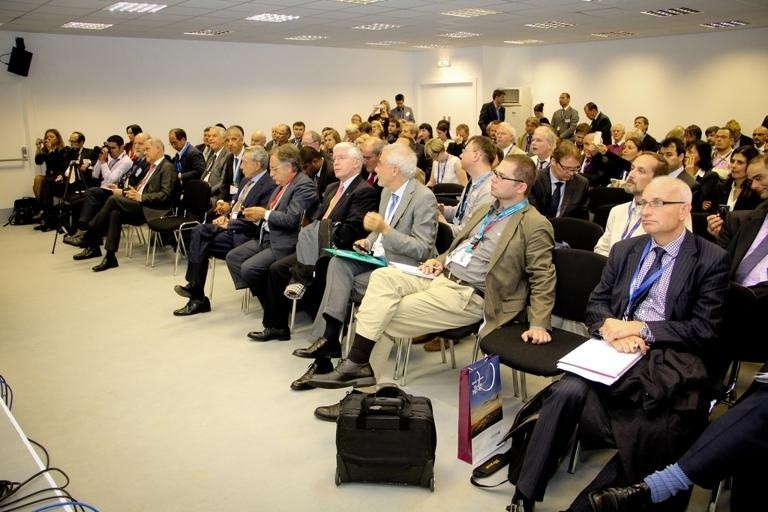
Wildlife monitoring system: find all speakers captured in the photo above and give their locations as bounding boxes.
[7,46,33,77]
[15,37,25,49]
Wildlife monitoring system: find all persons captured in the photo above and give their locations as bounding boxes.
[34,89,767,512]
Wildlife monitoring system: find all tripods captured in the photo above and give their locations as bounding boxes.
[51,161,88,254]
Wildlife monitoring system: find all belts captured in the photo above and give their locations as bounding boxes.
[441,268,484,299]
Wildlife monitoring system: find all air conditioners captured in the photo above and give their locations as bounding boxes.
[496,87,533,145]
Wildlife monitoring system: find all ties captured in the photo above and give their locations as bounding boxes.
[734,234,767,284]
[629,247,667,320]
[234,158,238,170]
[269,187,284,209]
[366,172,377,186]
[540,162,544,169]
[232,181,254,212]
[387,194,397,220]
[202,154,216,181]
[136,165,155,192]
[321,184,344,220]
[551,182,564,216]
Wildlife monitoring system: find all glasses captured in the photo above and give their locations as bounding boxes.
[637,199,685,207]
[557,160,582,171]
[492,170,524,183]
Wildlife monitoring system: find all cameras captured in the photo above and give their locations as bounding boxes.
[99,145,111,154]
[40,139,45,143]
[378,105,384,109]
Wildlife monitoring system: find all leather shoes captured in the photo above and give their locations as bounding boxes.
[174,283,204,299]
[174,296,210,315]
[74,244,101,260]
[507,498,535,512]
[314,391,341,422]
[587,483,645,511]
[308,358,377,388]
[293,336,342,358]
[424,336,459,351]
[92,257,118,270]
[63,229,89,245]
[291,362,334,391]
[248,328,291,341]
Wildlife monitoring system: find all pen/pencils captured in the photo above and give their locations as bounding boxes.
[633,343,639,349]
[418,262,440,270]
[352,243,369,253]
[241,205,245,210]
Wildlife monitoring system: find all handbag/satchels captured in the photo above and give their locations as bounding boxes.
[336,387,436,492]
[13,198,39,224]
[458,353,504,466]
[497,379,559,486]
[41,203,75,229]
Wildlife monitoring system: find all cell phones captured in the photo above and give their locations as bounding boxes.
[594,131,602,145]
[718,204,730,221]
